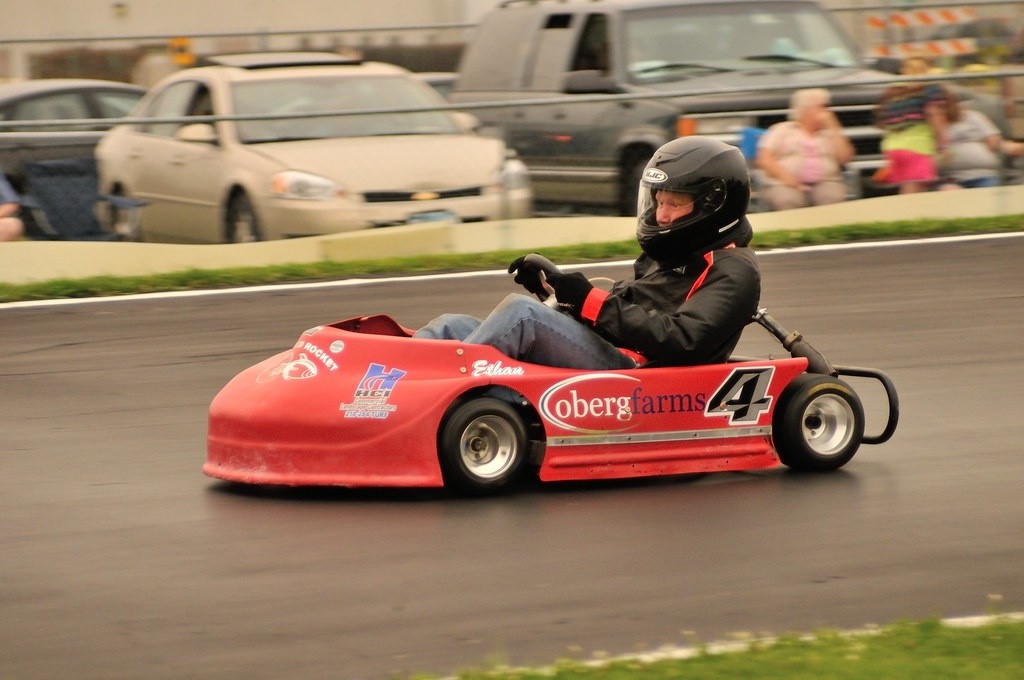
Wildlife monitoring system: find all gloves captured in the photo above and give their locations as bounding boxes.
[545,272,594,305]
[507,253,549,294]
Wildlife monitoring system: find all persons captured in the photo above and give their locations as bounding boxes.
[873,27,1024,195]
[0,175,25,241]
[402,136,760,369]
[749,87,854,215]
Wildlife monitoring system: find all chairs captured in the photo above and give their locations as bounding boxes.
[740,126,779,212]
[20,156,153,243]
[738,23,807,54]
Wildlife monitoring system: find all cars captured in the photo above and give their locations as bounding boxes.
[0,78,151,185]
[94,50,531,242]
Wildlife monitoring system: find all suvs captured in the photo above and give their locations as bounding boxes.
[445,0,953,218]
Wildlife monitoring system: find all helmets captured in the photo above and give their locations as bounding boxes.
[635,135,753,271]
